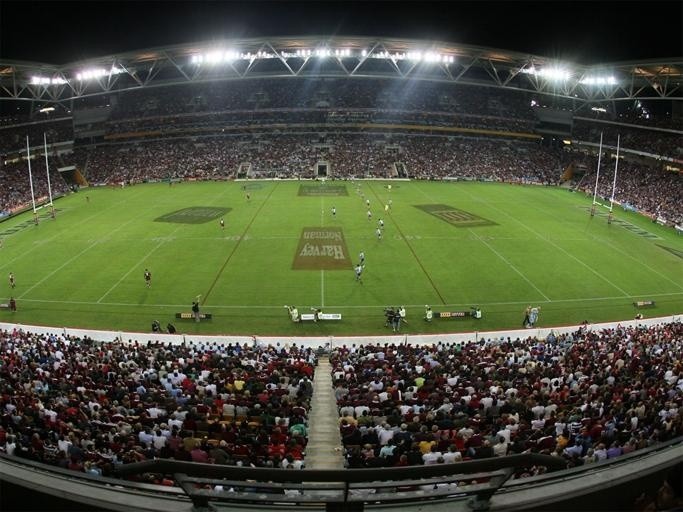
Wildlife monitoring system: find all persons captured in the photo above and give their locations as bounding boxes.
[0,250,682,505]
[0,77,682,244]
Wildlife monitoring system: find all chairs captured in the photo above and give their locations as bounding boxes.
[0,320,683,493]
